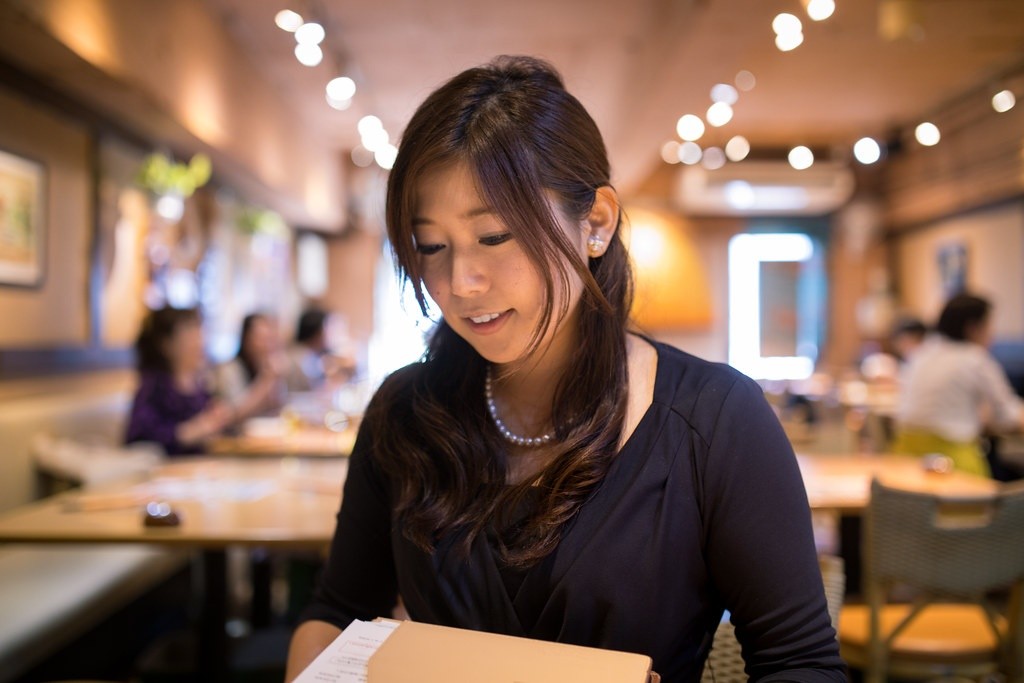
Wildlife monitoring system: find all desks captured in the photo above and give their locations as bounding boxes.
[0,456,348,636]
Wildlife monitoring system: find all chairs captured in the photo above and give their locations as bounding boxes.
[837,477,1024,683]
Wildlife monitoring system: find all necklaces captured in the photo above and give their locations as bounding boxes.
[484,359,580,447]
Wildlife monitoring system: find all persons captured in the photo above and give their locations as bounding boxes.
[286,303,373,396]
[121,303,285,460]
[210,307,309,418]
[883,290,1024,479]
[283,50,848,683]
